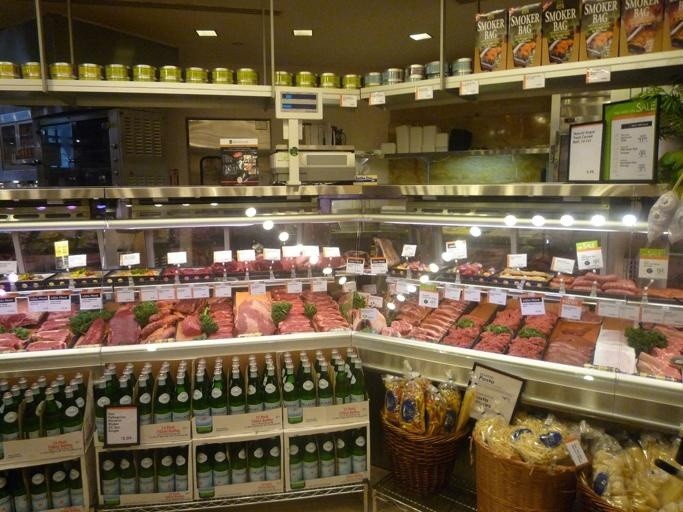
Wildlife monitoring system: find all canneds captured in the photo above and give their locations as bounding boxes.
[0,61,257,86]
[275,56,472,88]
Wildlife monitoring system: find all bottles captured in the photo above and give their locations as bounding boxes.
[0,461,83,512]
[98,446,189,495]
[281,345,366,424]
[288,426,369,483]
[0,370,85,438]
[193,353,281,434]
[91,360,192,442]
[195,436,282,489]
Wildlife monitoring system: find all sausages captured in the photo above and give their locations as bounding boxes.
[550,272,640,300]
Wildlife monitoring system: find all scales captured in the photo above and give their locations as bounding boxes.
[269,86,356,186]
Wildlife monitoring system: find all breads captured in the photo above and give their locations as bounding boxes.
[382,381,474,439]
[591,442,683,512]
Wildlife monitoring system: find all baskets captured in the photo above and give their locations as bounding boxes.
[475,417,592,512]
[581,452,683,512]
[381,412,471,495]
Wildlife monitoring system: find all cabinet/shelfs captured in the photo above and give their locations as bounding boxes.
[361,46,682,201]
[0,368,95,511]
[282,343,369,489]
[193,352,285,501]
[93,360,194,510]
[0,46,359,201]
